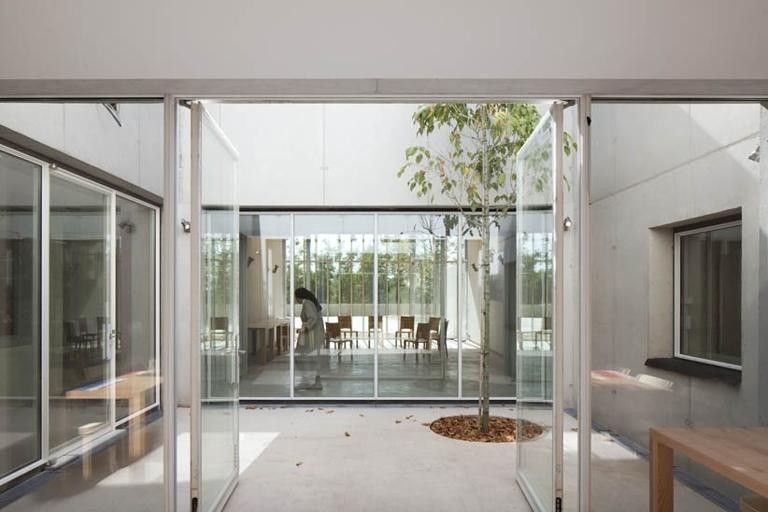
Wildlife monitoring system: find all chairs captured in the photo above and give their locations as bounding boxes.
[63,317,105,360]
[320,315,449,366]
[211,317,227,349]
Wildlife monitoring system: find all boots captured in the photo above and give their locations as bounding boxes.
[296,376,322,391]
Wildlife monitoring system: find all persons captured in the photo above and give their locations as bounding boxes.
[295,286,326,356]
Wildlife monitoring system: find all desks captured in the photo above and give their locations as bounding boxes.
[247,320,289,364]
[65,375,164,458]
[649,425,766,511]
